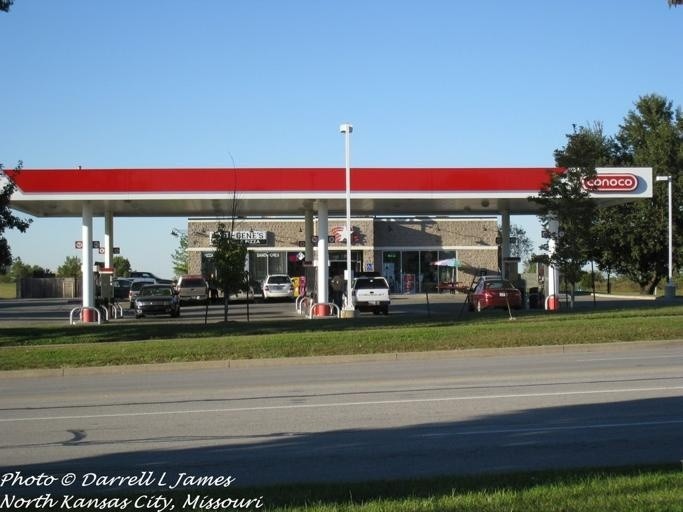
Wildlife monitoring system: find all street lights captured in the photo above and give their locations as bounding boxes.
[653,174,676,302]
[337,122,362,322]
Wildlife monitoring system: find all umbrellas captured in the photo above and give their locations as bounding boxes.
[428,257,463,284]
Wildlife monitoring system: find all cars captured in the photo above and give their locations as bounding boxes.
[108,269,534,318]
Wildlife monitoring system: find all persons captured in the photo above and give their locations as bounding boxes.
[328,268,345,315]
